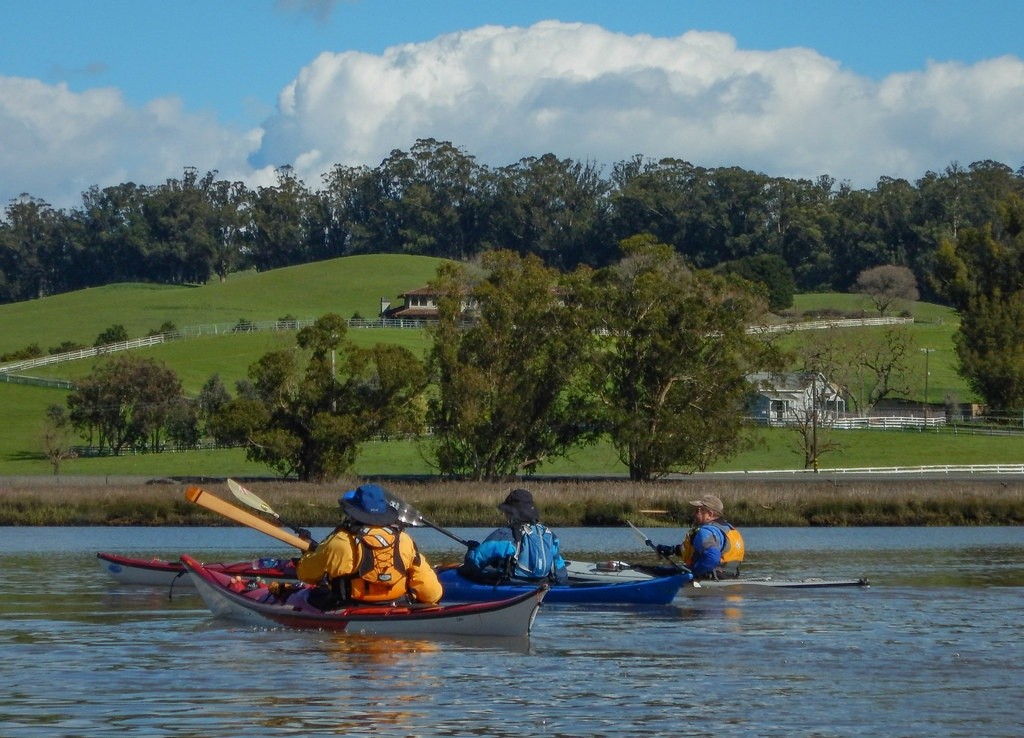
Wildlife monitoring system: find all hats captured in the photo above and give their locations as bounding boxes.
[498,489,540,520]
[344,489,356,499]
[689,495,723,513]
[338,485,399,527]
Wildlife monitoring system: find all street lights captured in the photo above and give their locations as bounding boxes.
[917,347,936,431]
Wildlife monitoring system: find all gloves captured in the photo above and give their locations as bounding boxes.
[657,544,675,557]
[468,540,481,550]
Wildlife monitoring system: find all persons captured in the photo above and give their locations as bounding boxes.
[657,495,744,580]
[286,484,443,609]
[464,489,569,586]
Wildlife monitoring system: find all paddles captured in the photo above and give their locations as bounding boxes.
[398,500,468,546]
[627,518,690,573]
[226,476,303,537]
[183,486,313,554]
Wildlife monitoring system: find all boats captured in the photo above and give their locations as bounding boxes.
[179,553,551,638]
[435,562,695,606]
[552,553,872,596]
[94,548,465,589]
